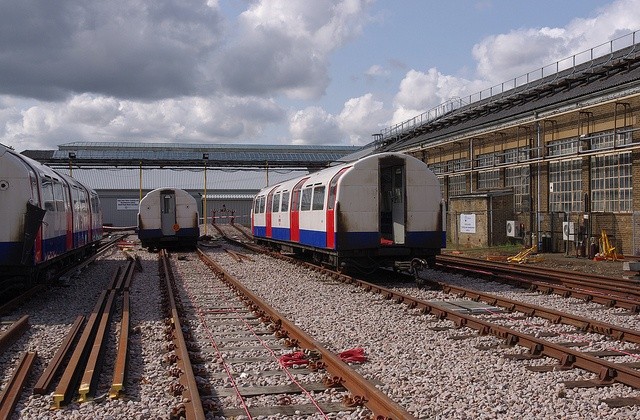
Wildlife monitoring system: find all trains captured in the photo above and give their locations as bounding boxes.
[251,152,447,275]
[136,187,202,252]
[0,142,102,288]
